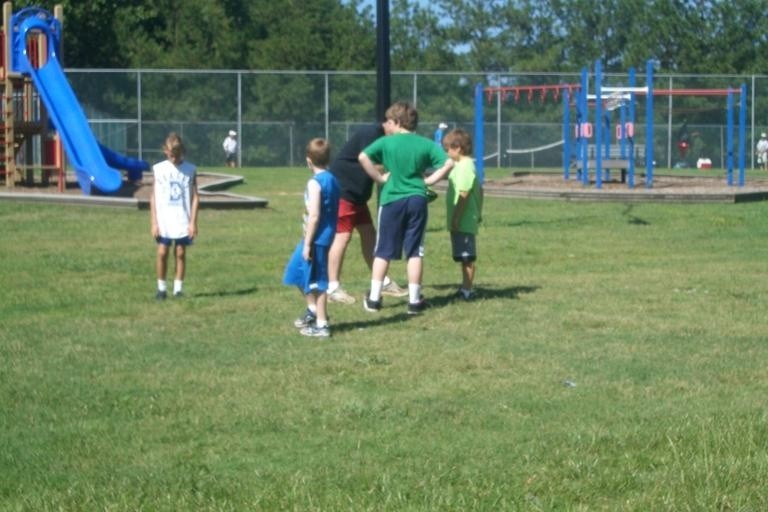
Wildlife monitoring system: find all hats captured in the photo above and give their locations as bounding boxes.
[438,122,448,129]
[228,129,237,137]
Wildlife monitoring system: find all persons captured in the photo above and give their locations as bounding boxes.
[329,109,409,305]
[434,122,448,146]
[441,129,484,300]
[223,130,237,168]
[756,133,768,171]
[147,133,199,298]
[282,138,339,337]
[358,103,454,315]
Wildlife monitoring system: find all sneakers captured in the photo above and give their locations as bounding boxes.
[293,305,320,328]
[380,279,410,298]
[172,291,186,298]
[363,288,384,313]
[326,286,357,305]
[445,287,476,302]
[298,323,331,338]
[406,298,437,315]
[155,289,168,300]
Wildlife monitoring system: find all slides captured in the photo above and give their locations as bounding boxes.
[19,16,122,194]
[96,141,151,180]
